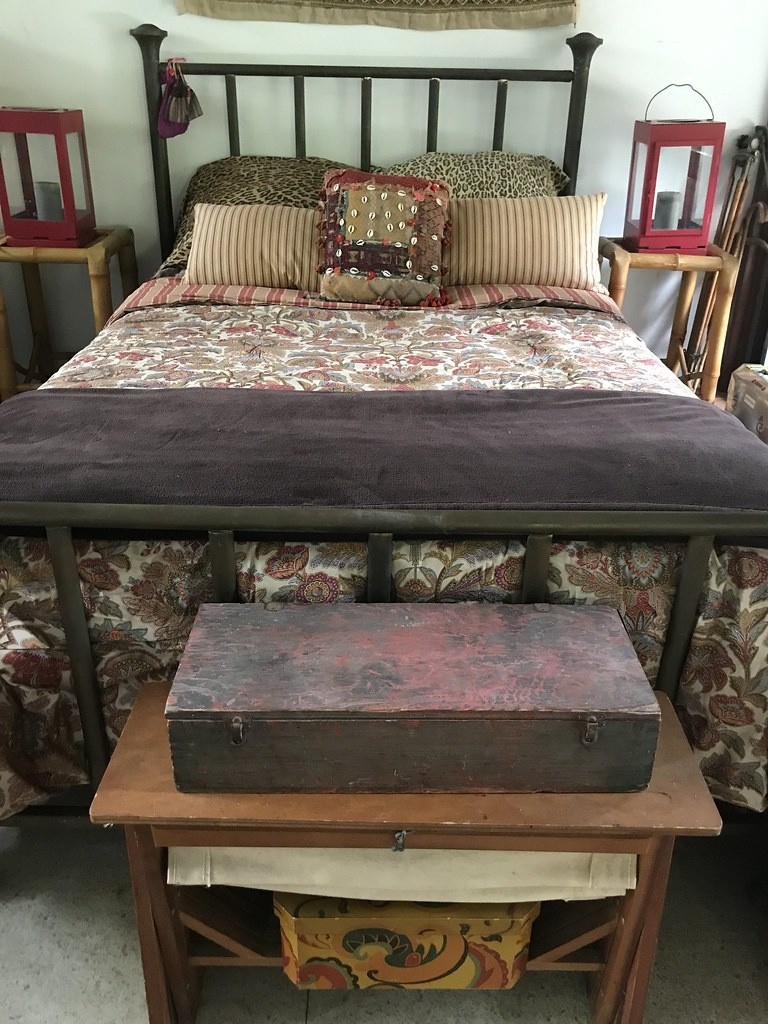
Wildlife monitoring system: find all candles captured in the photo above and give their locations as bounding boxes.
[653,190,682,231]
[33,182,63,221]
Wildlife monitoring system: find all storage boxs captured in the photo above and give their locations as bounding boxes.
[164,602,659,794]
[271,891,541,991]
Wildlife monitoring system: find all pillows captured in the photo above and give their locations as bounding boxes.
[367,151,571,221]
[441,192,610,294]
[181,202,322,293]
[317,167,456,308]
[153,154,366,277]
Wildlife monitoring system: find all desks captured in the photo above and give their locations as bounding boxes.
[86,685,723,1024]
[598,236,739,407]
[0,226,139,402]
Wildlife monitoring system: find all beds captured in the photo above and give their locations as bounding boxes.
[0,23,768,831]
